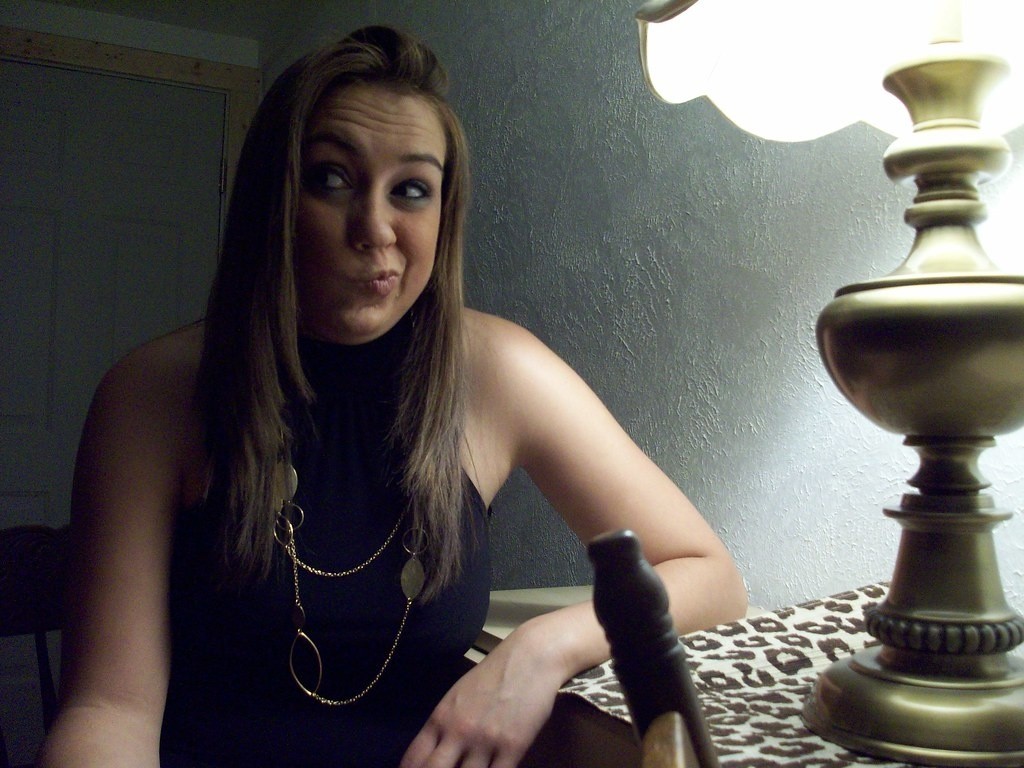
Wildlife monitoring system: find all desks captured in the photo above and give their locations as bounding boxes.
[464,584,914,768]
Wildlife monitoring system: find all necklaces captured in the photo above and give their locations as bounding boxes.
[277,441,429,708]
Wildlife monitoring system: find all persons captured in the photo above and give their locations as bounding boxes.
[44,21,751,768]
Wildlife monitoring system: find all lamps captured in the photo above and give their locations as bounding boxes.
[634,0,1024,768]
